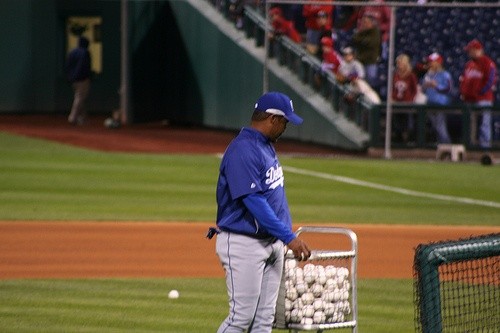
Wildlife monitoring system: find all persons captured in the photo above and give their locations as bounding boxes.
[211,0,500,149]
[63,36,94,126]
[216,90,313,333]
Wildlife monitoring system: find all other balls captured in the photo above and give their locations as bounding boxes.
[285,259,351,325]
[168,289,179,299]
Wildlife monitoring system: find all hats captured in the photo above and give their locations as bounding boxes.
[254,91,304,126]
[340,47,353,54]
[426,52,443,65]
[321,36,334,46]
[464,39,483,51]
[268,7,280,15]
